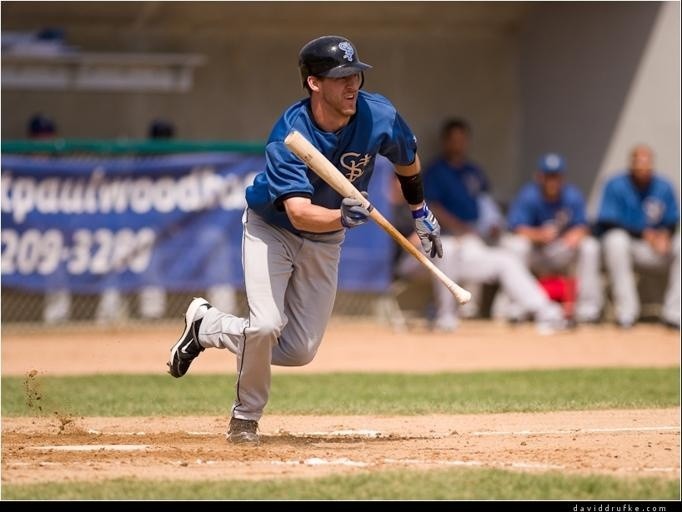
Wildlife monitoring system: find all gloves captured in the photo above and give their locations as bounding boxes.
[411,200,443,259]
[340,190,371,229]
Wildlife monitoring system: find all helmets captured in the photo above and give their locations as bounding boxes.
[297,35,373,89]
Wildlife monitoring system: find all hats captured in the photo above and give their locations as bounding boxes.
[538,152,567,173]
[440,112,468,128]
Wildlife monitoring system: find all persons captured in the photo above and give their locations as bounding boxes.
[387,168,566,333]
[423,119,534,323]
[489,153,608,326]
[595,142,681,329]
[138,120,244,320]
[22,112,129,322]
[165,33,444,444]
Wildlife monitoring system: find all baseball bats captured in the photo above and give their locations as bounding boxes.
[284,130,472,306]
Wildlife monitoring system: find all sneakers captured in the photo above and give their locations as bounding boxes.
[166,296,212,379]
[225,417,259,447]
